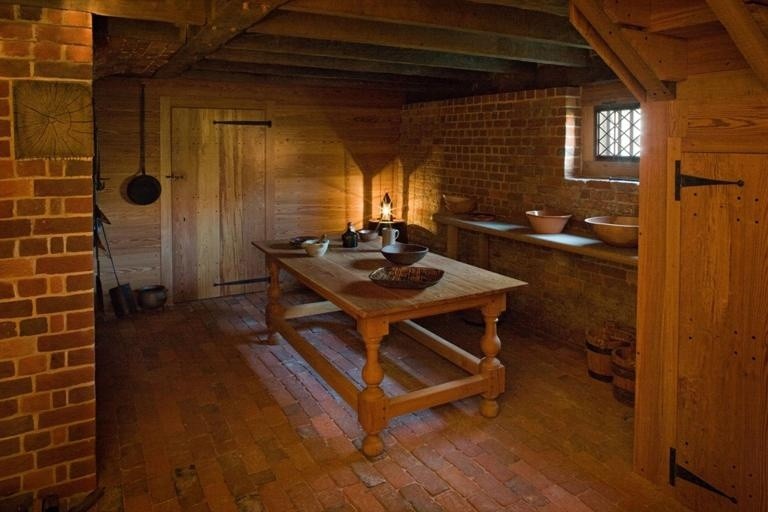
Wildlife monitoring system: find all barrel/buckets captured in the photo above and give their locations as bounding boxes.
[609,342,639,410]
[584,322,633,383]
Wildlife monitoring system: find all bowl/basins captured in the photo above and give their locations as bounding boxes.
[525,208,573,234]
[302,239,329,256]
[583,215,639,249]
[441,194,476,215]
[356,230,379,241]
[381,244,429,265]
[289,236,321,249]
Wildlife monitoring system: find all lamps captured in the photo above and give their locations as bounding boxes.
[377,191,397,222]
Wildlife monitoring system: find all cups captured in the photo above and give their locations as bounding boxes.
[381,227,400,245]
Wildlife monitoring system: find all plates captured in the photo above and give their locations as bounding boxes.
[369,266,445,289]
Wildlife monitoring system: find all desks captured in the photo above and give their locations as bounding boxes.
[433,208,638,328]
[250,229,530,458]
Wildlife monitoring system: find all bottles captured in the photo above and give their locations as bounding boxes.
[342,222,358,248]
